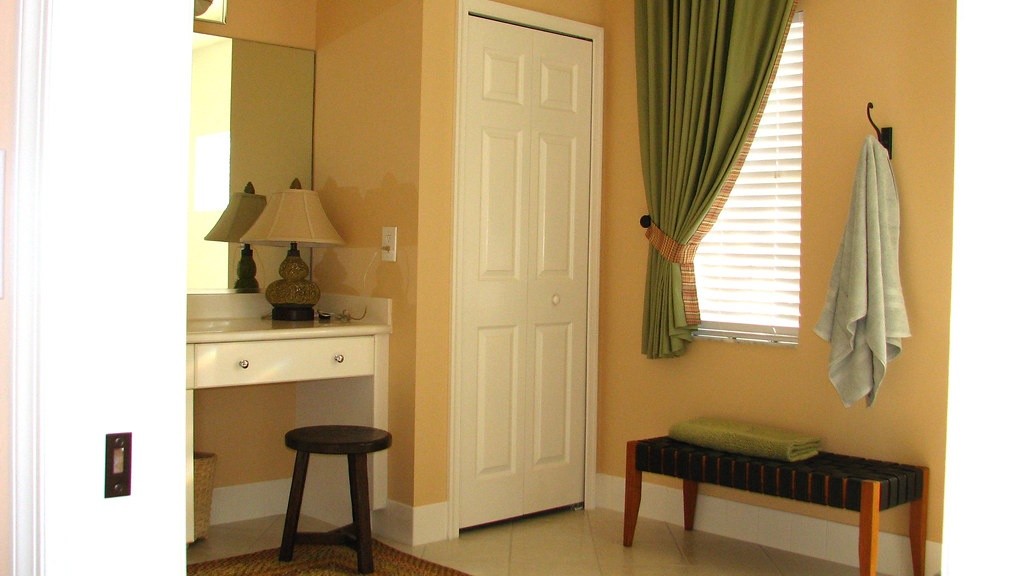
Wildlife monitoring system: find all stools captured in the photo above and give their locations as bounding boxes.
[277,424,395,576]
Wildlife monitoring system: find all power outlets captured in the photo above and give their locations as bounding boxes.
[381,226,399,263]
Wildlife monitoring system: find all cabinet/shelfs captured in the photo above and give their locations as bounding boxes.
[186,292,391,553]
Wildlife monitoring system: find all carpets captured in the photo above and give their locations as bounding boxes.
[187,535,475,576]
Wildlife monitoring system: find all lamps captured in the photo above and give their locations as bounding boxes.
[201,180,267,295]
[239,176,391,322]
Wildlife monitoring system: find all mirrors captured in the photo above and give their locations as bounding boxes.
[186,31,317,296]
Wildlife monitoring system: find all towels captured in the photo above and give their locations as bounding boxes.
[811,134,912,411]
[668,415,827,464]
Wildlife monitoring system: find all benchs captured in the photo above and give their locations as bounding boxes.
[623,433,931,576]
